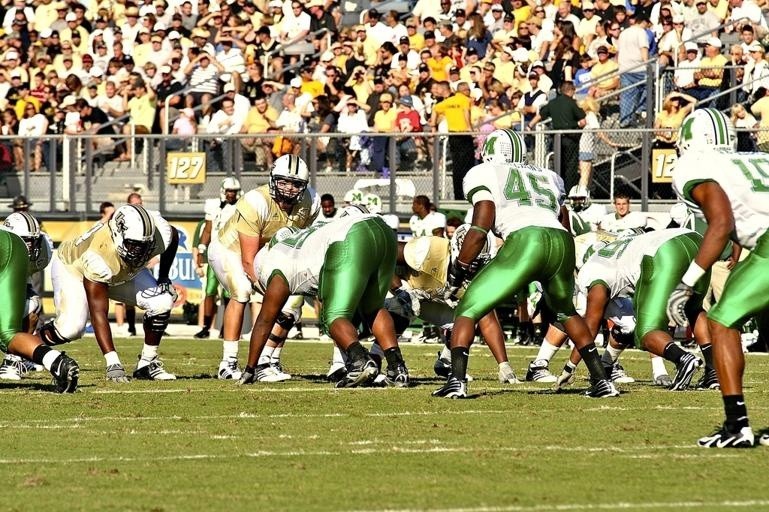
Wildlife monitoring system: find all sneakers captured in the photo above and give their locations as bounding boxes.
[292,333,303,338]
[134,355,177,381]
[695,367,721,390]
[526,361,558,383]
[698,420,769,448]
[0,350,79,393]
[432,357,474,399]
[194,330,209,338]
[326,353,410,387]
[218,358,291,383]
[668,353,702,390]
[746,341,769,353]
[579,364,635,398]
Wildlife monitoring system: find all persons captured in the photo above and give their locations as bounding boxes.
[0,2,769,397]
[667,108,769,448]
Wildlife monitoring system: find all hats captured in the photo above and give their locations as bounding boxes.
[638,10,653,28]
[684,37,722,51]
[749,46,762,52]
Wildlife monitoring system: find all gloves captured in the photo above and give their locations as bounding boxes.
[666,257,706,327]
[653,374,671,386]
[555,360,576,386]
[443,284,460,310]
[103,351,129,384]
[498,362,522,384]
[158,278,179,303]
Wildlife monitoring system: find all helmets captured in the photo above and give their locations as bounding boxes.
[450,222,491,281]
[220,177,242,203]
[268,225,299,250]
[268,154,309,211]
[108,204,155,268]
[676,108,737,157]
[568,184,591,211]
[584,240,610,263]
[479,128,527,163]
[344,189,382,214]
[2,211,44,261]
